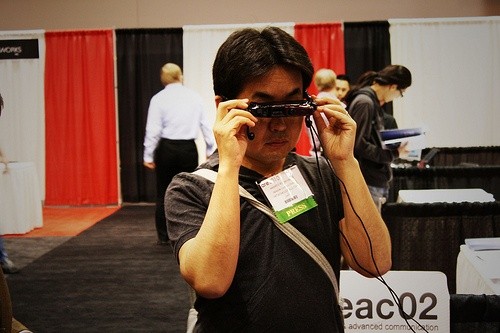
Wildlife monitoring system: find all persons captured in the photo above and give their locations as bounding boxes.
[0,92,24,275]
[302,68,348,158]
[162,25,393,333]
[334,73,352,101]
[357,70,404,163]
[143,61,218,248]
[344,65,412,223]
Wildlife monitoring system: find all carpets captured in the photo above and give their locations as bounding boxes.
[5,206,195,333]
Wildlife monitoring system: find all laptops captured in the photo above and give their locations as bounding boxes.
[404,147,439,168]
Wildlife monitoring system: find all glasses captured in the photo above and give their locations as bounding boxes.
[397,88,405,97]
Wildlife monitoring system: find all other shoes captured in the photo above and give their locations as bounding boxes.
[160,239,170,243]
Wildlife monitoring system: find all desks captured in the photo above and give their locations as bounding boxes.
[0,162,43,236]
[395,189,496,204]
[456,244,500,296]
